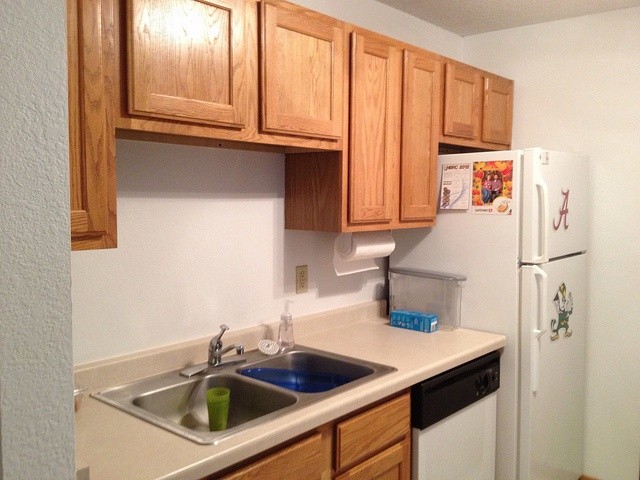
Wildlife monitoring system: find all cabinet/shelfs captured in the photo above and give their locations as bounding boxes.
[115,1,351,151]
[444,56,514,150]
[220,394,411,480]
[285,23,441,234]
[67,0,118,254]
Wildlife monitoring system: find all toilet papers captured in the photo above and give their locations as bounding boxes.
[332,233,396,277]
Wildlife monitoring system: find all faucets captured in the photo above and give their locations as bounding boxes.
[206,323,243,368]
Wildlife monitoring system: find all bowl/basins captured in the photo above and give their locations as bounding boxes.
[241,364,354,393]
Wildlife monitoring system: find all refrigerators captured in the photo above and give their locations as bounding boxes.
[388,148,591,480]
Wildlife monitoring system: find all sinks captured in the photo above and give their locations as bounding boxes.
[233,341,398,398]
[89,371,309,446]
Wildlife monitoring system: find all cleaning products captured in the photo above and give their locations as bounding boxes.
[280,301,296,351]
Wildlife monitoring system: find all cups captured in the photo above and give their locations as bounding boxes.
[206,387,230,431]
